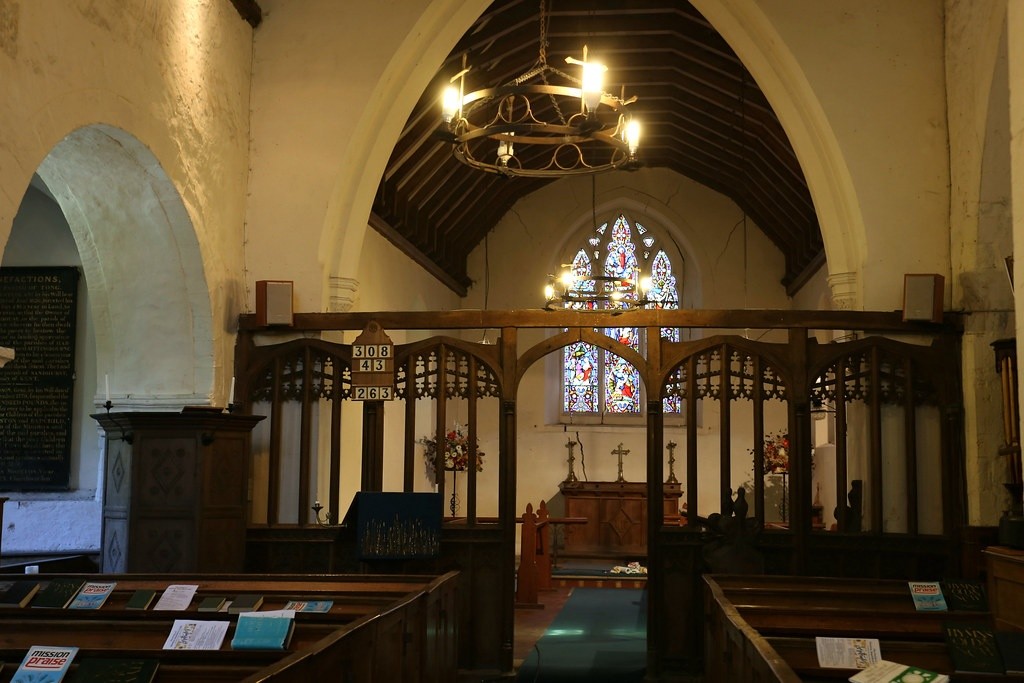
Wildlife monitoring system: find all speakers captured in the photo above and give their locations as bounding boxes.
[902,274,945,324]
[256,280,294,327]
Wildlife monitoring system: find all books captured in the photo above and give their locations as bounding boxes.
[69,580,118,608]
[0,580,40,608]
[280,600,334,614]
[0,660,6,673]
[230,609,296,651]
[122,587,158,610]
[227,594,266,615]
[197,597,226,611]
[71,656,164,683]
[848,659,951,683]
[8,644,80,683]
[994,632,1024,675]
[942,580,990,611]
[32,576,88,608]
[908,580,949,612]
[941,621,1004,677]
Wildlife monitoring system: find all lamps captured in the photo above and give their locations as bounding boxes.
[440,0,641,179]
[541,176,680,310]
[811,401,836,420]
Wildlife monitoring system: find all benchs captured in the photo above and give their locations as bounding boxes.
[700,572,1024,683]
[0,572,463,683]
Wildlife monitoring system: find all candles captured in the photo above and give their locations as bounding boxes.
[230,376,235,404]
[105,372,110,401]
